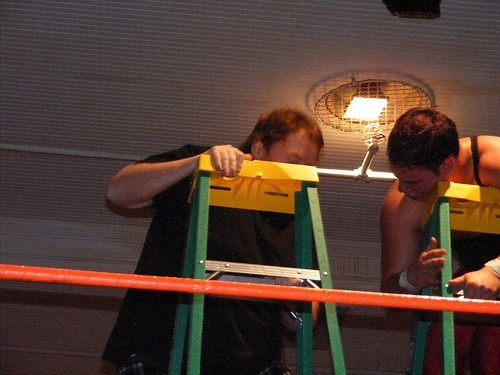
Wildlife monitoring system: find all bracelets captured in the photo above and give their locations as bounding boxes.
[484,258,500,277]
[399,265,420,293]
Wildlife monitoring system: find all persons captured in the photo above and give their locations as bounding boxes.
[101,109,329,374]
[377,107,500,373]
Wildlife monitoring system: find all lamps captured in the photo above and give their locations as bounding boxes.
[305,70,434,136]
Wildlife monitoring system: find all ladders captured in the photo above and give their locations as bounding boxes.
[170,154,346,375]
[407,182,499,375]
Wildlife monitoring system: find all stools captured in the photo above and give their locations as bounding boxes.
[169,151,348,375]
[417,179,500,375]
